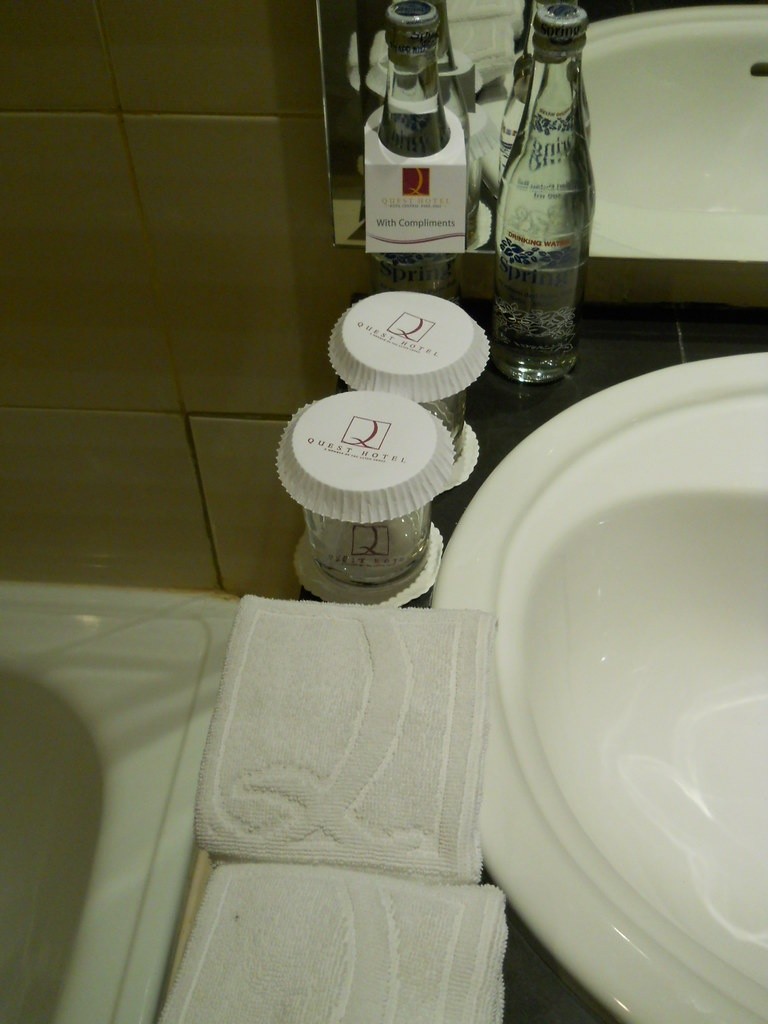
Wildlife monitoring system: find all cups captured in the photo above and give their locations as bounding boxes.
[302,502,431,590]
[349,385,466,464]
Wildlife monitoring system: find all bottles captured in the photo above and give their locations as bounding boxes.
[499,0,591,187]
[425,0,471,208]
[370,0,459,305]
[489,3,596,385]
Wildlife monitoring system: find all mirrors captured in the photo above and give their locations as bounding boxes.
[318,0,768,265]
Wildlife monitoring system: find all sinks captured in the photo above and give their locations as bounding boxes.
[426,346,767,1024]
[458,4,768,262]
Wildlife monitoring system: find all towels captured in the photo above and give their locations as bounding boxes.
[156,863,509,1024]
[192,591,500,886]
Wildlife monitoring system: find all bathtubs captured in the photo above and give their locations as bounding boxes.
[0,578,240,1024]
